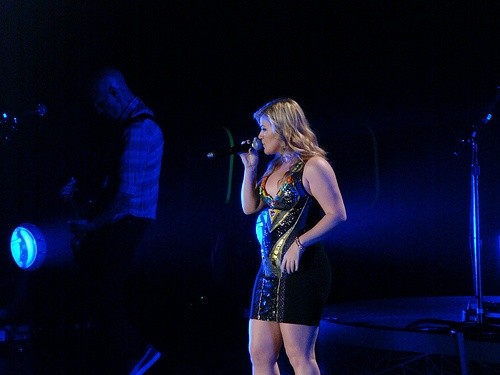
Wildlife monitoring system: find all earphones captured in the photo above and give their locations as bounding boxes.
[110,87,118,98]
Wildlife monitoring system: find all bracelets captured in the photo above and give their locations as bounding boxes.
[295,236,306,252]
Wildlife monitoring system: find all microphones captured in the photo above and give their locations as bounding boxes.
[224,138,262,156]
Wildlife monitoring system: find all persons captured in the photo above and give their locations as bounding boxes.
[236,98,347,374]
[66,69,164,375]
